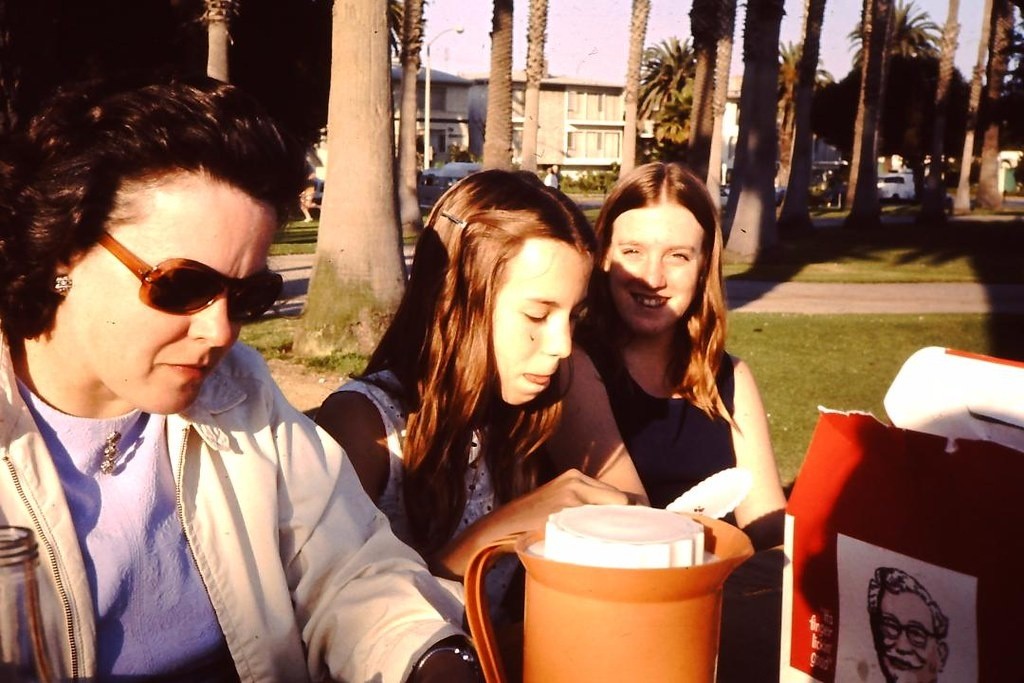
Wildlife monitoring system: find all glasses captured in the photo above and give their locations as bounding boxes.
[84,220,283,325]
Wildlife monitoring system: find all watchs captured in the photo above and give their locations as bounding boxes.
[415,644,479,679]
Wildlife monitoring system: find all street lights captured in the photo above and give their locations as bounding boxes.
[424,25,464,185]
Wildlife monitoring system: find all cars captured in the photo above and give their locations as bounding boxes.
[418,162,483,204]
[876,172,916,201]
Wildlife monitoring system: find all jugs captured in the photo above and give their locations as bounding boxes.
[464,502,756,683]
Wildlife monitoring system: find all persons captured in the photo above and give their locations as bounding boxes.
[300,151,323,222]
[543,164,562,190]
[1013,156,1024,192]
[571,162,788,563]
[830,154,849,205]
[0,75,482,683]
[917,173,947,224]
[315,170,652,637]
